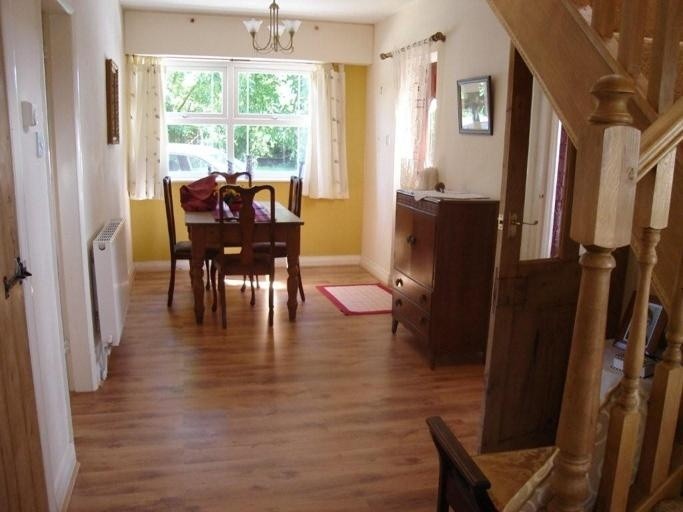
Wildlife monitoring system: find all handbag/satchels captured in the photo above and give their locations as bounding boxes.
[179,175,218,212]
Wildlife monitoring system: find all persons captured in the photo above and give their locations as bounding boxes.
[625,308,652,345]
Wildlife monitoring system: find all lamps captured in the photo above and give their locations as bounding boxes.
[241,0,302,54]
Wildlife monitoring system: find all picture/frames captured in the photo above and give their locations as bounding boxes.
[106,60,118,145]
[456,75,492,135]
[612,290,668,356]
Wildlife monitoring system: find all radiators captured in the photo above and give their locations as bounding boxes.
[93,217,130,347]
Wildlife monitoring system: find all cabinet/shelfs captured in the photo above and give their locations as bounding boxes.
[391,194,500,371]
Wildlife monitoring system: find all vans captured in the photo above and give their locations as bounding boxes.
[169,143,246,181]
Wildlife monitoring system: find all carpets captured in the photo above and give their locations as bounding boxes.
[316,283,393,315]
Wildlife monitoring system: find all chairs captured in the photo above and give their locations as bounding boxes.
[427,373,653,510]
[163,172,305,329]
